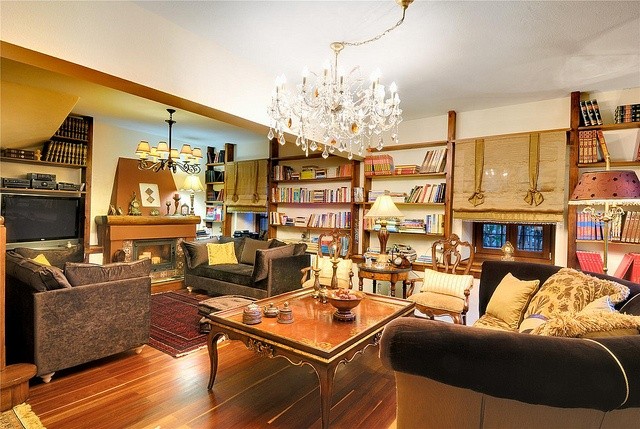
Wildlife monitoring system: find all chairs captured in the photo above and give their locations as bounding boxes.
[405,232,475,325]
[300,228,355,289]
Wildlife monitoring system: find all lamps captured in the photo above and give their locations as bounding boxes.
[179,176,204,217]
[569,170,640,275]
[135,108,203,174]
[362,195,405,255]
[263,0,413,161]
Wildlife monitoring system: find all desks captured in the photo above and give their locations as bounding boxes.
[358,262,413,299]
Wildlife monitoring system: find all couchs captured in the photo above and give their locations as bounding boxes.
[6,247,153,384]
[379,260,640,428]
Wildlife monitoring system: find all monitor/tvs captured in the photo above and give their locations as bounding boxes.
[0,193,84,250]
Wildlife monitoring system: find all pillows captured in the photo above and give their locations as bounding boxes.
[251,244,296,282]
[485,273,541,331]
[313,256,353,290]
[32,253,51,266]
[206,241,239,266]
[267,239,307,255]
[239,236,274,265]
[523,267,632,319]
[420,268,474,300]
[531,295,638,338]
[220,234,245,257]
[181,237,219,270]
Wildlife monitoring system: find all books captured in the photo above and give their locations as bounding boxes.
[271,186,351,202]
[575,251,605,275]
[585,100,596,125]
[269,211,350,227]
[614,103,640,123]
[284,238,334,254]
[592,130,597,162]
[583,131,589,164]
[368,189,408,203]
[205,170,224,181]
[273,164,352,180]
[419,148,448,173]
[208,191,218,201]
[196,230,210,240]
[630,212,640,242]
[407,183,446,203]
[217,191,221,201]
[630,253,640,284]
[614,253,633,280]
[207,151,224,163]
[363,213,445,235]
[414,255,438,264]
[620,211,631,242]
[597,129,610,160]
[4,148,35,155]
[220,189,224,201]
[364,154,394,176]
[52,116,90,142]
[580,100,590,127]
[215,206,223,221]
[579,131,584,163]
[588,131,593,163]
[42,140,89,165]
[364,247,396,258]
[577,211,622,243]
[394,164,420,175]
[634,218,640,243]
[591,99,602,125]
[625,211,636,242]
[5,153,34,160]
[207,207,215,221]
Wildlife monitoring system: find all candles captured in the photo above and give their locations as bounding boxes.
[373,66,382,85]
[337,76,345,94]
[389,82,398,100]
[274,78,281,101]
[372,81,376,102]
[322,58,331,85]
[301,67,309,92]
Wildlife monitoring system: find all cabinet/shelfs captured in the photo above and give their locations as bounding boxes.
[567,92,640,286]
[269,137,359,258]
[205,142,232,239]
[362,110,456,272]
[1,111,94,265]
[94,215,202,296]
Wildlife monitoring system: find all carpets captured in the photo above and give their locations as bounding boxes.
[1,405,44,427]
[150,288,230,358]
